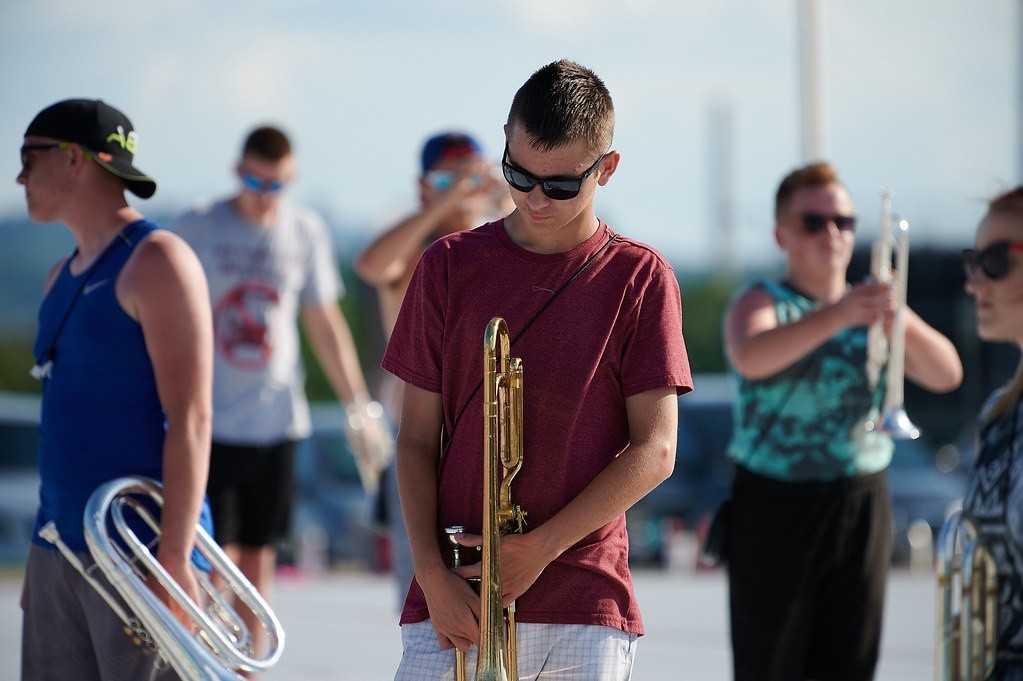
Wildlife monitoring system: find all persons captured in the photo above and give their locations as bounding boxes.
[357,131,516,612]
[16,98,214,680]
[722,163,964,681]
[382,58,693,681]
[168,125,393,681]
[960,186,1023,681]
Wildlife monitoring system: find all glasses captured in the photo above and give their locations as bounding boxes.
[21,142,92,166]
[425,170,486,192]
[243,171,282,193]
[962,241,1023,280]
[502,140,610,200]
[783,213,855,233]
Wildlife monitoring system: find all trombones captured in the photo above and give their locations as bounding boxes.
[454,315,526,680]
[938,501,998,680]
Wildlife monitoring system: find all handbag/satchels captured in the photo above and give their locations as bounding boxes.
[703,498,733,564]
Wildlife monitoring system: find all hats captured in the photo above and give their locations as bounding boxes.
[25,99,156,199]
[422,133,483,176]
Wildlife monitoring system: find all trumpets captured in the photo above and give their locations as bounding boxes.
[36,476,285,680]
[868,188,920,440]
